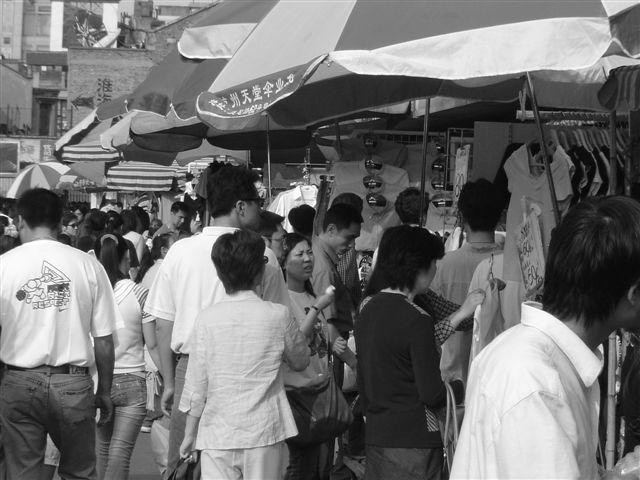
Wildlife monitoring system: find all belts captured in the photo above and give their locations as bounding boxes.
[0,362,88,374]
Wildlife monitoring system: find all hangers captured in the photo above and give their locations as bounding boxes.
[333,128,454,215]
[512,111,627,171]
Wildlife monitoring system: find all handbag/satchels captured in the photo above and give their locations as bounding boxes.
[285,375,354,448]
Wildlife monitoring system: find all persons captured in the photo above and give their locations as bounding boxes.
[353,180,640,479]
[1,141,365,480]
